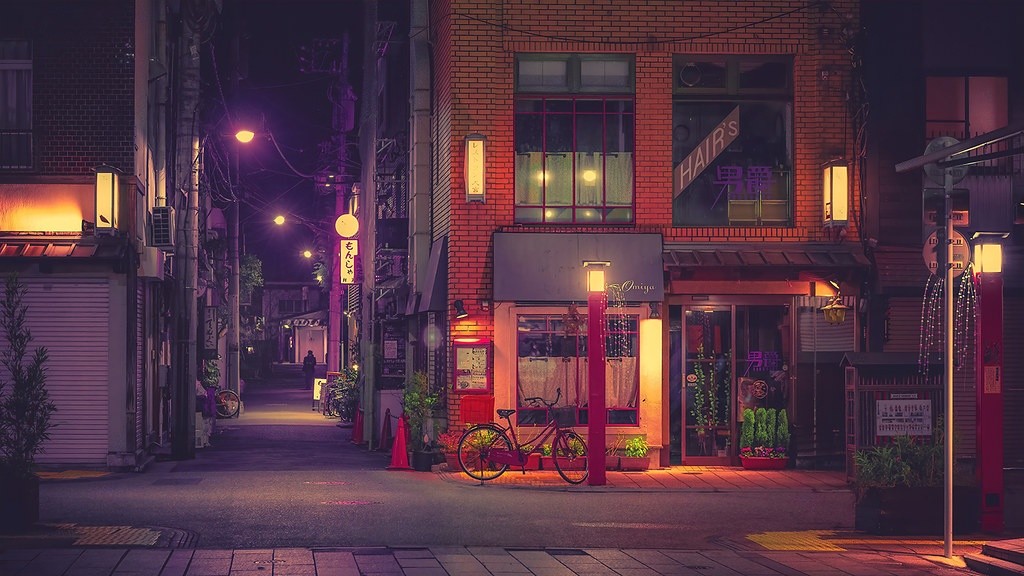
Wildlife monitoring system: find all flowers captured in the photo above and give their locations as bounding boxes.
[738,407,790,459]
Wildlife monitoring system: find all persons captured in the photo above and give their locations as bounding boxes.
[303,350,316,389]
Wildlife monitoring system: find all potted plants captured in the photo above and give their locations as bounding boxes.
[618,436,651,472]
[538,434,587,471]
[605,433,625,471]
[844,412,978,535]
[0,269,59,532]
[398,367,453,472]
[438,420,540,472]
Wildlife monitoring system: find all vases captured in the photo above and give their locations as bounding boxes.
[738,455,790,473]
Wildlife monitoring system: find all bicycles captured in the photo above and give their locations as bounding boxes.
[324,377,353,422]
[456,387,589,486]
[214,386,240,419]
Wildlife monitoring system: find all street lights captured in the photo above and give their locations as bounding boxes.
[582,259,612,486]
[970,168,1013,533]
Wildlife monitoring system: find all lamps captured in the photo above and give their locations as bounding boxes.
[814,280,851,326]
[89,163,119,239]
[454,300,468,319]
[463,132,486,203]
[822,157,851,244]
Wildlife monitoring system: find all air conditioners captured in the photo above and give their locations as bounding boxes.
[151,207,175,251]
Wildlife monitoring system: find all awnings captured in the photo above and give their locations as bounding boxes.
[492,232,664,301]
[418,236,448,312]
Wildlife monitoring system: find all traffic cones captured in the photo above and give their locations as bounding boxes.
[349,402,368,445]
[383,414,416,470]
[373,409,392,452]
